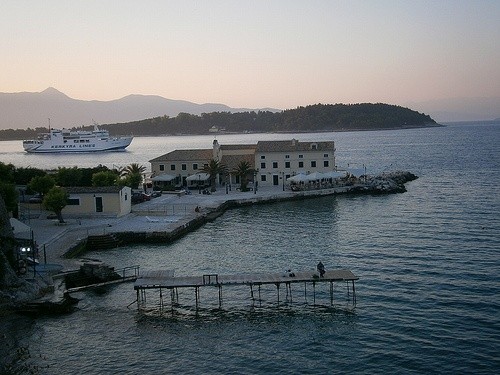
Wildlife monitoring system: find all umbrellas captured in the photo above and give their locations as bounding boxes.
[288,171,346,191]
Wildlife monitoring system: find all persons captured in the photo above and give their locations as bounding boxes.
[195,205,202,213]
[317,260,327,277]
[148,190,162,198]
[183,188,212,196]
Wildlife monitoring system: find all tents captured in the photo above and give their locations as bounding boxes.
[9,217,34,262]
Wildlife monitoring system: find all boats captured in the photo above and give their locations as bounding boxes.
[22,127,133,153]
[49,260,122,290]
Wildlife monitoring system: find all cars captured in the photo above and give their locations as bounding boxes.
[141,194,150,201]
